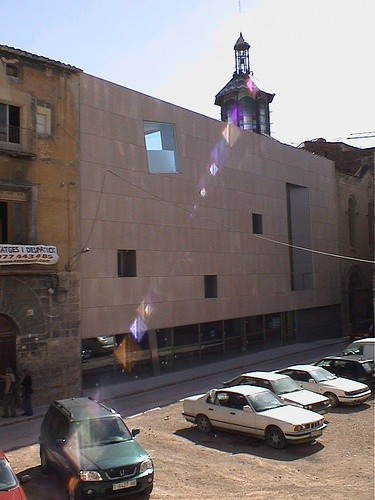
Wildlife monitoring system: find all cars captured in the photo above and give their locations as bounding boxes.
[274,363,372,409]
[181,384,327,450]
[222,371,332,416]
[1,449,32,500]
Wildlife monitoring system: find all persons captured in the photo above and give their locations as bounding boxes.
[2,367,16,419]
[18,369,34,417]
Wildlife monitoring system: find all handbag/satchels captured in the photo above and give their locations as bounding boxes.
[7,375,16,394]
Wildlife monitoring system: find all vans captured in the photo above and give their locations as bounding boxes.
[341,338,375,356]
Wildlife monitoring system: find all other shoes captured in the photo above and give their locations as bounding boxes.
[22,413,33,416]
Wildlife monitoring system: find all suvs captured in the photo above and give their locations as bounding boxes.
[40,397,155,500]
[313,356,375,394]
